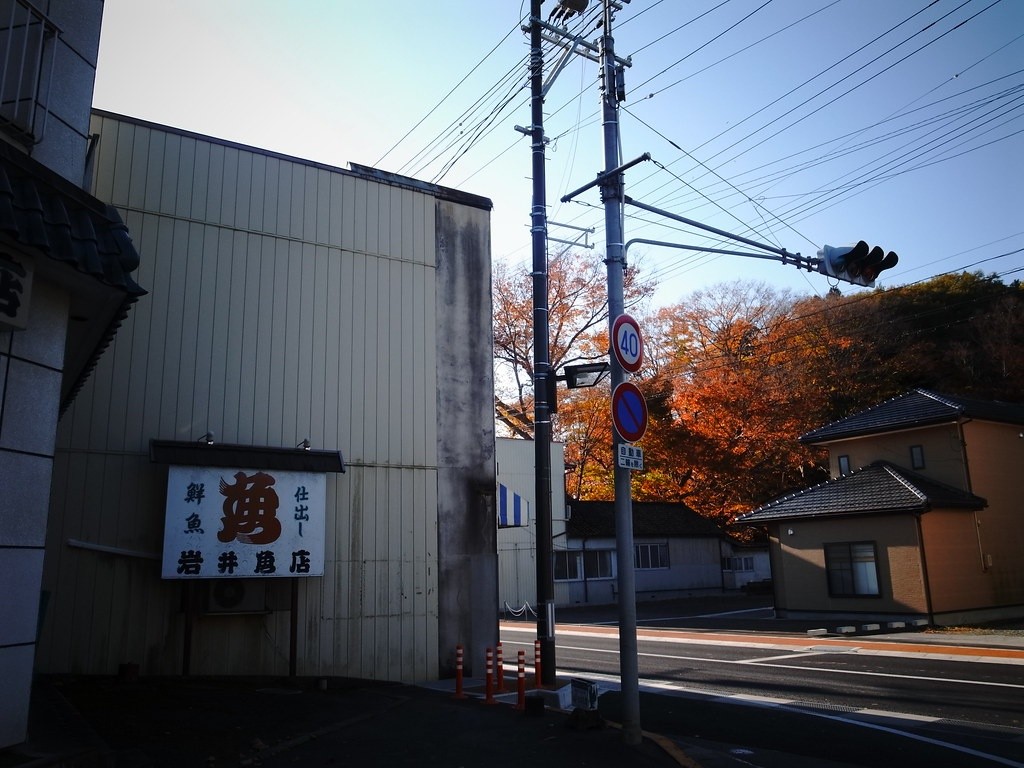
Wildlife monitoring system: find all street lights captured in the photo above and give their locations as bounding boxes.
[533,359,610,683]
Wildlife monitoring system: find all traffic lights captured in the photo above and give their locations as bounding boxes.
[818,240,899,287]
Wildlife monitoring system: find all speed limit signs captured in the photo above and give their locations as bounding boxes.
[612,314,647,372]
